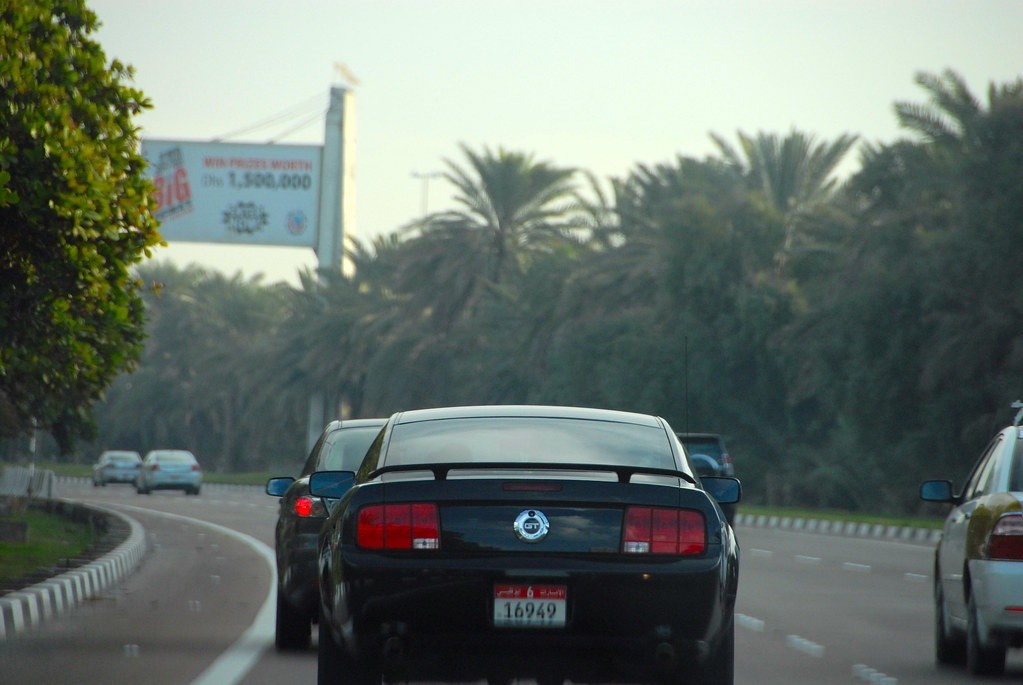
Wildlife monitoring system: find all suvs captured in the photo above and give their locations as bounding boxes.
[675,433,737,532]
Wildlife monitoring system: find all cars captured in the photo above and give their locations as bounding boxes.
[309,404,746,682]
[95,450,143,485]
[134,450,204,494]
[919,403,1023,677]
[261,419,391,654]
